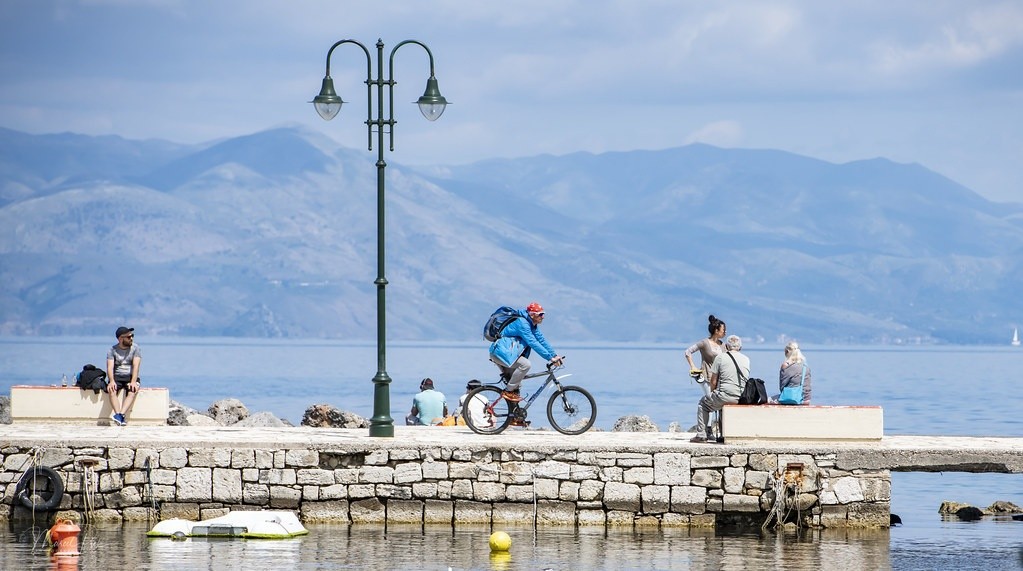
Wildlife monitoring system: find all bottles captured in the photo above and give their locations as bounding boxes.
[62,376,68,388]
[72,376,77,386]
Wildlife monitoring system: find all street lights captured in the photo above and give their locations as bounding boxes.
[308,37,452,437]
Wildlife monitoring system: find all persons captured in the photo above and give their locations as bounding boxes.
[768,343,811,405]
[406,378,448,426]
[685,315,750,443]
[459,380,497,427]
[489,303,564,426]
[107,326,142,425]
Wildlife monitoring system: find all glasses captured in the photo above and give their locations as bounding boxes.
[121,334,134,340]
[535,313,545,316]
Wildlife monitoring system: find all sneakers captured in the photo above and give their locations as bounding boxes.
[113,413,127,426]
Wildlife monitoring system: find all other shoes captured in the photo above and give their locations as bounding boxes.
[509,419,527,426]
[501,389,523,401]
[689,433,716,443]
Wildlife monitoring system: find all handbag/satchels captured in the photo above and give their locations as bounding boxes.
[777,385,804,405]
[738,378,768,406]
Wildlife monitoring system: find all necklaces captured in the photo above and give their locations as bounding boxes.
[711,340,719,350]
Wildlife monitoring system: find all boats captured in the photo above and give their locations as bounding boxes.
[146,510,309,538]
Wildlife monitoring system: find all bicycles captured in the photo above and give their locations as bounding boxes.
[463,356,597,436]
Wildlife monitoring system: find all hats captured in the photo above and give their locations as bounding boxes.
[115,327,134,339]
[527,302,543,313]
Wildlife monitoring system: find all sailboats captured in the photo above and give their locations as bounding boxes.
[1012,329,1021,347]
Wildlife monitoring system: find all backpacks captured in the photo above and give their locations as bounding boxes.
[482,305,532,343]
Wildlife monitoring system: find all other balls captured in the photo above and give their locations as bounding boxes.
[489,531,511,550]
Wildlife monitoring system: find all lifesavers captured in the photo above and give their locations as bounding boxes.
[16,466,64,513]
